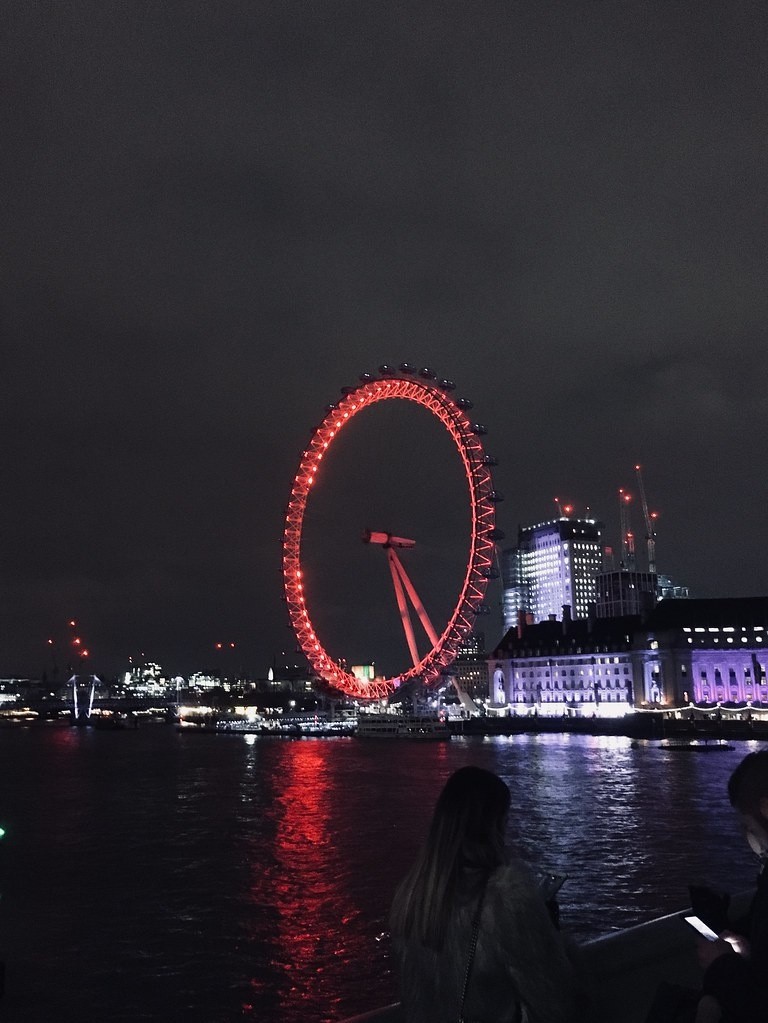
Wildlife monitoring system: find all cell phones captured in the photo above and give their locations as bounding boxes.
[539,871,566,901]
[684,915,720,942]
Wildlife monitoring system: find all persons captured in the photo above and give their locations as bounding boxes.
[693,748,768,1023]
[444,716,450,731]
[391,765,575,1023]
